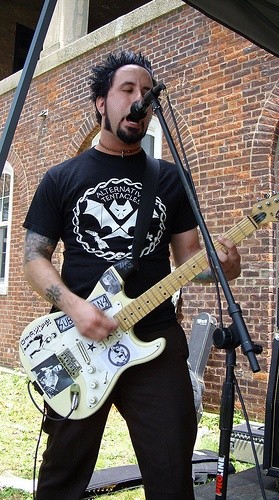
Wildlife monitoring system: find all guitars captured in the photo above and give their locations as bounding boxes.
[18,190,279,420]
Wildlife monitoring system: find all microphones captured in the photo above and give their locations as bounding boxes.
[130,83,166,119]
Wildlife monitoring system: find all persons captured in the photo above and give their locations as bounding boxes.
[23,49,241,500]
